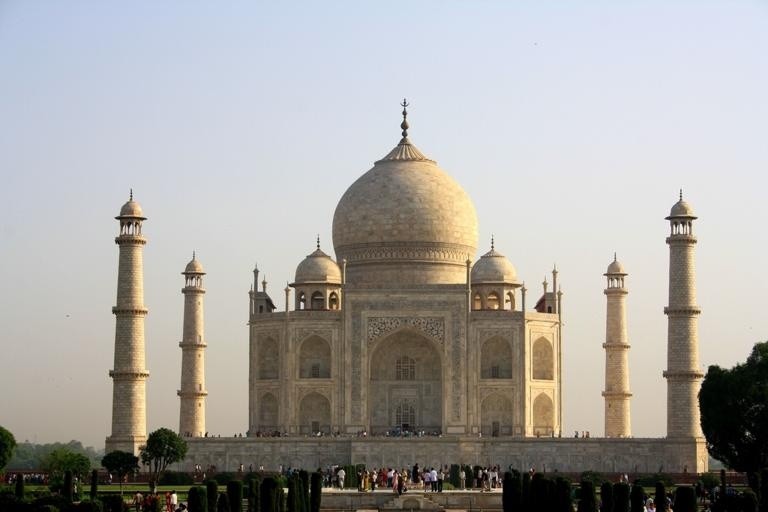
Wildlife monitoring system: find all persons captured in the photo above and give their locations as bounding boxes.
[179,425,443,437]
[696,482,700,502]
[639,493,676,511]
[701,486,706,503]
[199,463,201,471]
[6,471,50,486]
[624,471,629,484]
[131,489,179,512]
[727,483,734,496]
[180,505,189,512]
[107,472,112,484]
[206,463,216,472]
[713,484,720,501]
[249,463,253,472]
[240,463,244,473]
[618,472,624,483]
[260,463,264,472]
[176,502,184,511]
[71,481,80,502]
[195,463,198,471]
[272,458,503,495]
